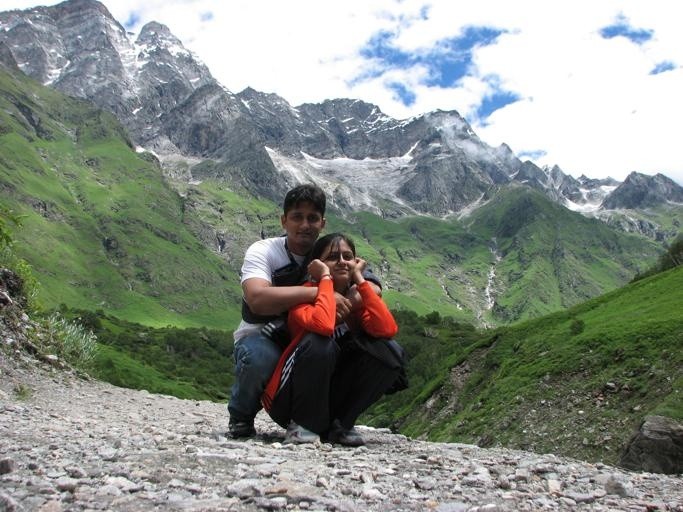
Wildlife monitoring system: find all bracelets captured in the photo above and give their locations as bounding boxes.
[320,273,333,280]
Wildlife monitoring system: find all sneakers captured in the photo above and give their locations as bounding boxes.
[328,418,366,446]
[283,423,320,443]
[229,411,256,436]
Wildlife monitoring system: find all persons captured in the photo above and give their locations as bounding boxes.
[227,183,384,439]
[259,231,410,445]
